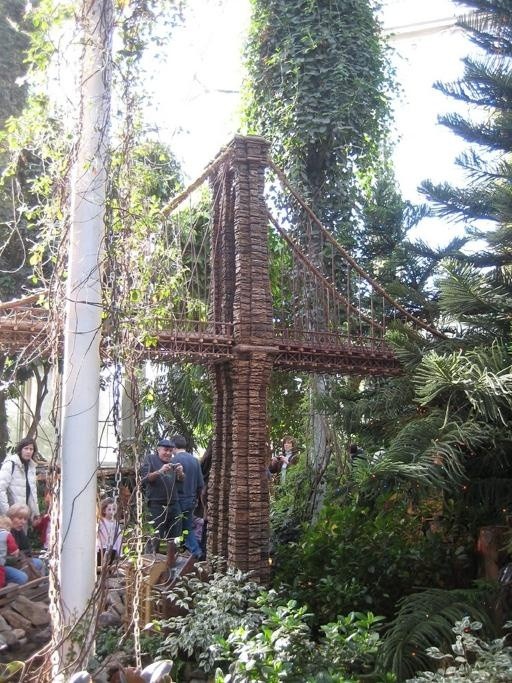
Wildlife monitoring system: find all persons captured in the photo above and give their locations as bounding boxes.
[269,436,301,490]
[348,439,370,464]
[135,435,211,561]
[0,438,123,590]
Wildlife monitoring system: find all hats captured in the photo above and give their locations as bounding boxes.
[157,439,175,448]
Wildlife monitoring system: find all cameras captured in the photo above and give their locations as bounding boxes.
[169,464,177,471]
[276,457,282,461]
